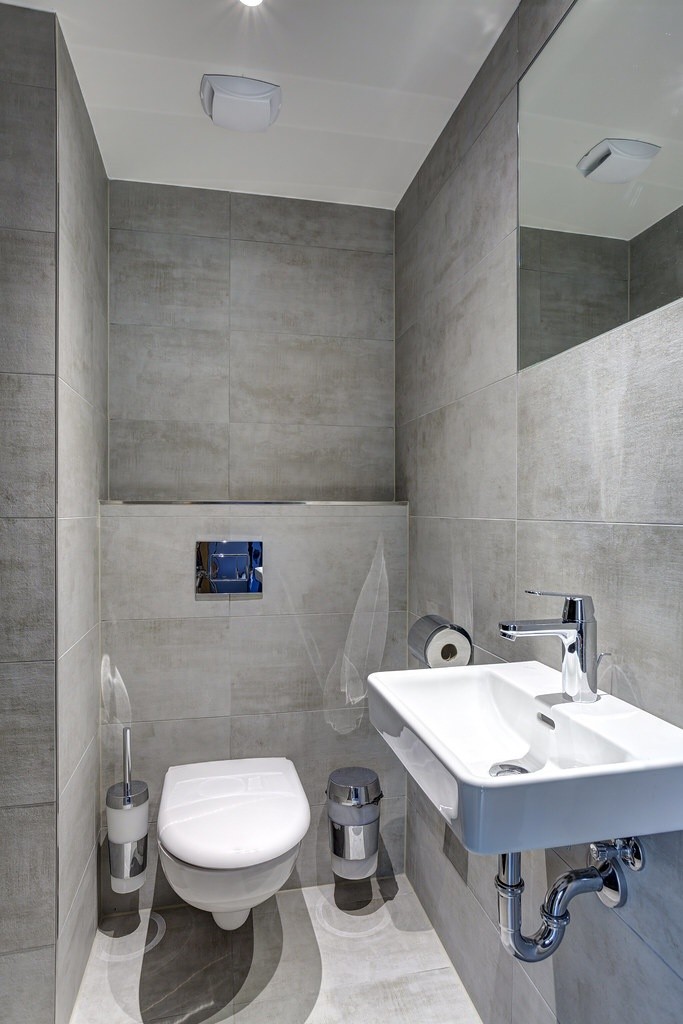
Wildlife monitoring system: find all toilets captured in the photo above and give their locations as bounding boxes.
[155,757,313,932]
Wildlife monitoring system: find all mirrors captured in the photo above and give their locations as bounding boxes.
[516,2,681,376]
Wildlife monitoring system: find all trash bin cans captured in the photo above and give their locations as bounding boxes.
[324,767,384,880]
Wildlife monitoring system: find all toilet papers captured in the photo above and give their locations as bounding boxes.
[406,614,474,668]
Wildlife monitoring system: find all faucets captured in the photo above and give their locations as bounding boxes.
[497,589,604,704]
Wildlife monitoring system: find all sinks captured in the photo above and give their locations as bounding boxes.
[367,659,683,856]
